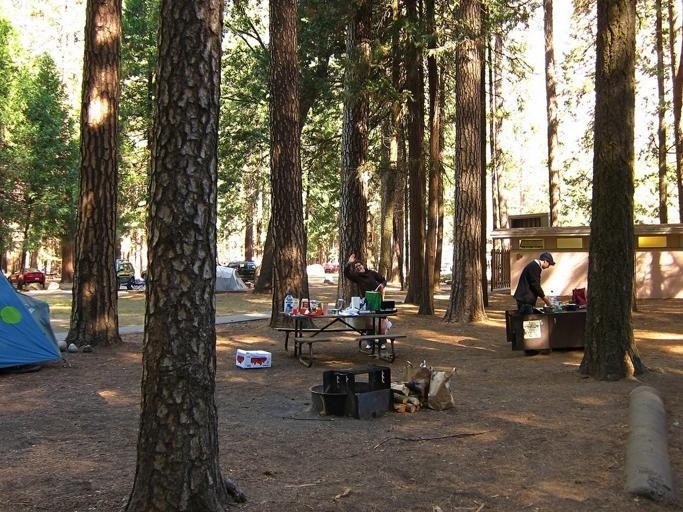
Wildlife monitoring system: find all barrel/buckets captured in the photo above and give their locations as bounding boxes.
[365,291,381,311]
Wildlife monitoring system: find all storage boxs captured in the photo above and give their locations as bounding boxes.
[235,348,272,369]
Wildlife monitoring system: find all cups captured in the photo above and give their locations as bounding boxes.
[327,308,340,315]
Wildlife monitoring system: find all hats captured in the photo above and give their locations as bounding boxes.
[540,251,556,266]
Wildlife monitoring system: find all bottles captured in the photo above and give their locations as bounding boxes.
[284,293,323,315]
[351,297,360,310]
[549,290,556,305]
[337,299,343,311]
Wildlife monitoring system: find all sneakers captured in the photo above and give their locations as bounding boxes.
[380,344,387,351]
[365,344,371,349]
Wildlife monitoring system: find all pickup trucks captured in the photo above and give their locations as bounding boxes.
[224,261,259,283]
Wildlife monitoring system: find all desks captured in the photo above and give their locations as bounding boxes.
[281,310,398,357]
[505,303,587,352]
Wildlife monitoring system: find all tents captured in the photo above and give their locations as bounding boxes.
[214,266,249,293]
[0,268,63,369]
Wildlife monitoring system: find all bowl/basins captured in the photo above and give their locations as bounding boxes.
[564,304,577,311]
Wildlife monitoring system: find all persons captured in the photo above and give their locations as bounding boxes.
[344,253,387,350]
[513,250,555,354]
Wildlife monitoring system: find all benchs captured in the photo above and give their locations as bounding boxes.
[274,327,377,355]
[293,335,407,367]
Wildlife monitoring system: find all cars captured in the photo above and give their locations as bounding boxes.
[323,261,340,274]
[7,267,45,288]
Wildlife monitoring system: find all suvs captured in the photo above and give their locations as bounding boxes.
[117,258,135,290]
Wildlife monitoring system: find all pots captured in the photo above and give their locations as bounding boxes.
[542,306,556,313]
